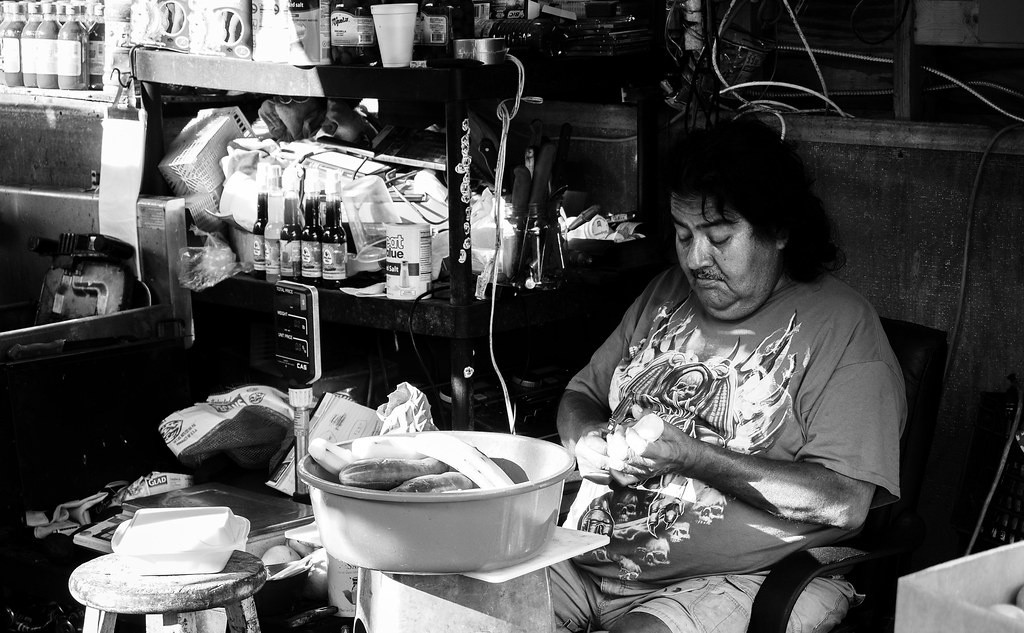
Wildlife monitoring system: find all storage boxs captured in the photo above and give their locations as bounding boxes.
[460,80,644,236]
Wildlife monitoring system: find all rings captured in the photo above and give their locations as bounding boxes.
[600,461,606,470]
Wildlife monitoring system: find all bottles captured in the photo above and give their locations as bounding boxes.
[265,164,283,281]
[0,0,105,90]
[280,168,300,281]
[322,172,348,289]
[301,169,321,287]
[253,163,267,280]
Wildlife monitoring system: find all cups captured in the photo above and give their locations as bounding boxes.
[342,175,402,263]
[421,0,474,59]
[505,203,566,292]
[371,4,418,68]
[331,0,377,64]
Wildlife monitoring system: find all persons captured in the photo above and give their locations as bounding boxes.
[547,126,908,633]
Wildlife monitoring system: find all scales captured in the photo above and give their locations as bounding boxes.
[72,278,323,568]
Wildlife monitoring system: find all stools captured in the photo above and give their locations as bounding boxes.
[69,549,267,633]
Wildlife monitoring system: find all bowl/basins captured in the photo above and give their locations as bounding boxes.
[296,435,576,572]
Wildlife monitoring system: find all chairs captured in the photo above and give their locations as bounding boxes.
[530,260,957,633]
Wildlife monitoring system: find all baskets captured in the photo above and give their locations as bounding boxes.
[158,106,256,213]
[177,193,222,233]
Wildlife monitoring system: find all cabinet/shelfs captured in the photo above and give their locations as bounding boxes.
[134,42,577,432]
